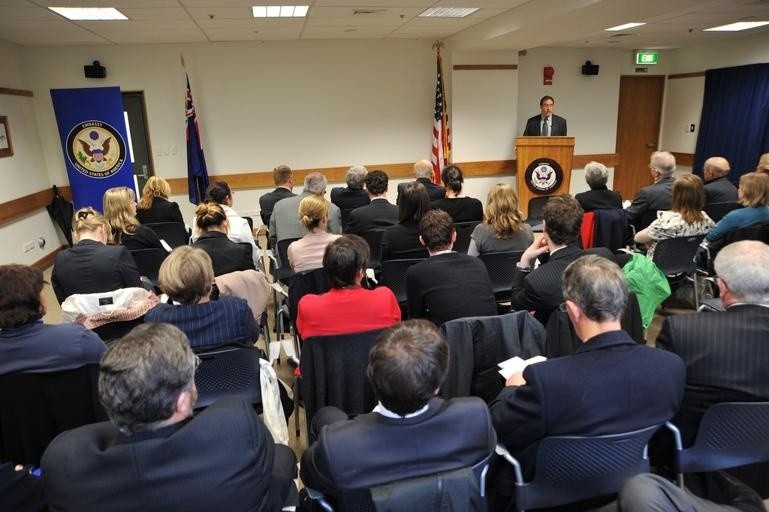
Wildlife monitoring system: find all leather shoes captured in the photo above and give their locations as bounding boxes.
[713,473,766,512]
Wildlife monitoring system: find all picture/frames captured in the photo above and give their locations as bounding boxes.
[0,115,13,158]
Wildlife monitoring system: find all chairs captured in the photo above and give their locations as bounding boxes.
[188,337,266,419]
[1,362,114,469]
[298,445,499,510]
[289,326,396,440]
[98,443,300,511]
[497,422,660,510]
[662,400,767,509]
[46,203,767,356]
[436,309,546,405]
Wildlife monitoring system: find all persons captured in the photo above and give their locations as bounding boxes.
[260,158,687,503]
[574,149,768,512]
[523,96,567,136]
[1,176,299,512]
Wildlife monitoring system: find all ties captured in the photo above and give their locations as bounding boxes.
[543,118,549,135]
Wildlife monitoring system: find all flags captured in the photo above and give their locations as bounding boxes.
[430,54,451,187]
[185,78,210,205]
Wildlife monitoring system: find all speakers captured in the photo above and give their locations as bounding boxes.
[582,64,599,76]
[83,65,105,78]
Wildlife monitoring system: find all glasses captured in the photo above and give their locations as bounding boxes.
[559,297,577,312]
